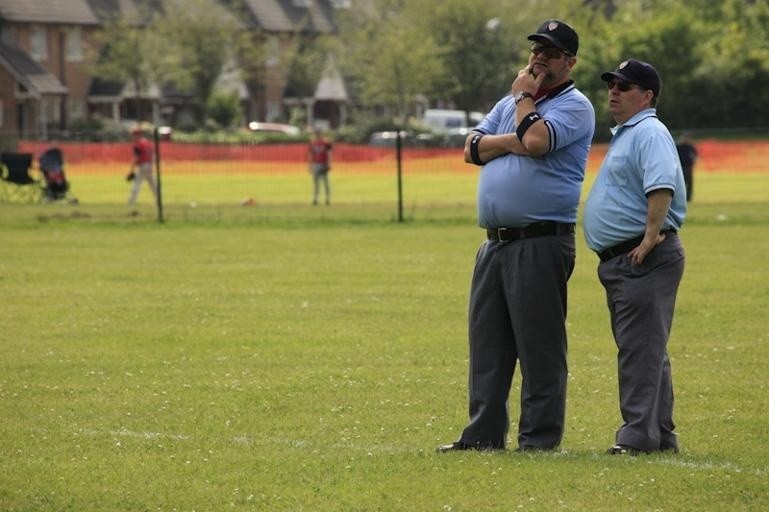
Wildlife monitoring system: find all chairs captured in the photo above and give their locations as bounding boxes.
[1,151,41,204]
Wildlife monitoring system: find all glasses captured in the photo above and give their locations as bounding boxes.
[529,40,564,59]
[604,78,640,93]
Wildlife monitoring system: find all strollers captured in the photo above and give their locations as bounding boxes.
[38,147,79,206]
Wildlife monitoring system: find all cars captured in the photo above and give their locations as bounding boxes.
[122,119,172,138]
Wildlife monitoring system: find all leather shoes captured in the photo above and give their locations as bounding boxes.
[437,440,505,460]
[605,444,646,459]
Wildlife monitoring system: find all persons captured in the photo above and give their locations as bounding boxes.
[438,21,595,452]
[676,133,699,202]
[583,60,686,454]
[306,127,333,205]
[125,128,157,205]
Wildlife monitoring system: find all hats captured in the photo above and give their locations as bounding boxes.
[599,57,660,96]
[526,18,580,56]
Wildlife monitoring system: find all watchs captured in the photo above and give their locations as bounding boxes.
[514,91,536,105]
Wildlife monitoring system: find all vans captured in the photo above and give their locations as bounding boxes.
[249,121,299,138]
[423,110,485,132]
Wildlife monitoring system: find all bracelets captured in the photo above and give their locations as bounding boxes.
[517,113,540,140]
[470,133,484,167]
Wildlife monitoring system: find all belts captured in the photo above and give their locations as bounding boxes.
[486,221,577,242]
[595,227,677,265]
